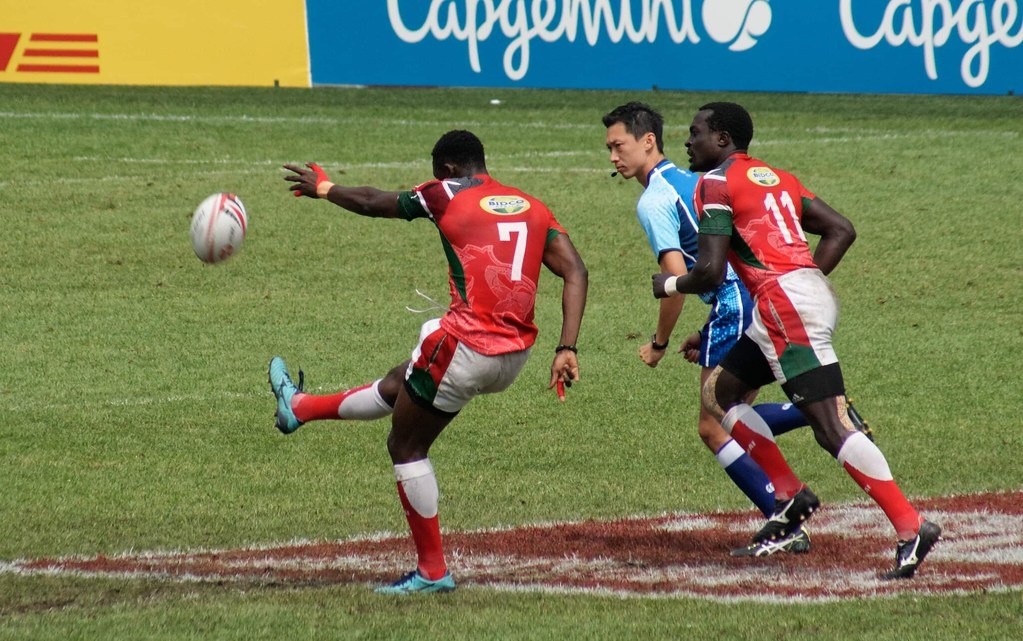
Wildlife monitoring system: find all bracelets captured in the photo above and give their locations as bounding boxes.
[555,344,578,354]
[652,333,669,350]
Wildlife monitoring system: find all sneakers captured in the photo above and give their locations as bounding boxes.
[876,521,943,578]
[267,356,304,434]
[730,526,809,557]
[846,397,873,442]
[752,482,821,542]
[374,566,455,596]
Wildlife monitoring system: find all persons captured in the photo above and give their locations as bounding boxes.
[651,101,941,579]
[602,101,811,557]
[267,128,589,595]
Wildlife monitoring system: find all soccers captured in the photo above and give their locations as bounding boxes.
[189,191,247,262]
